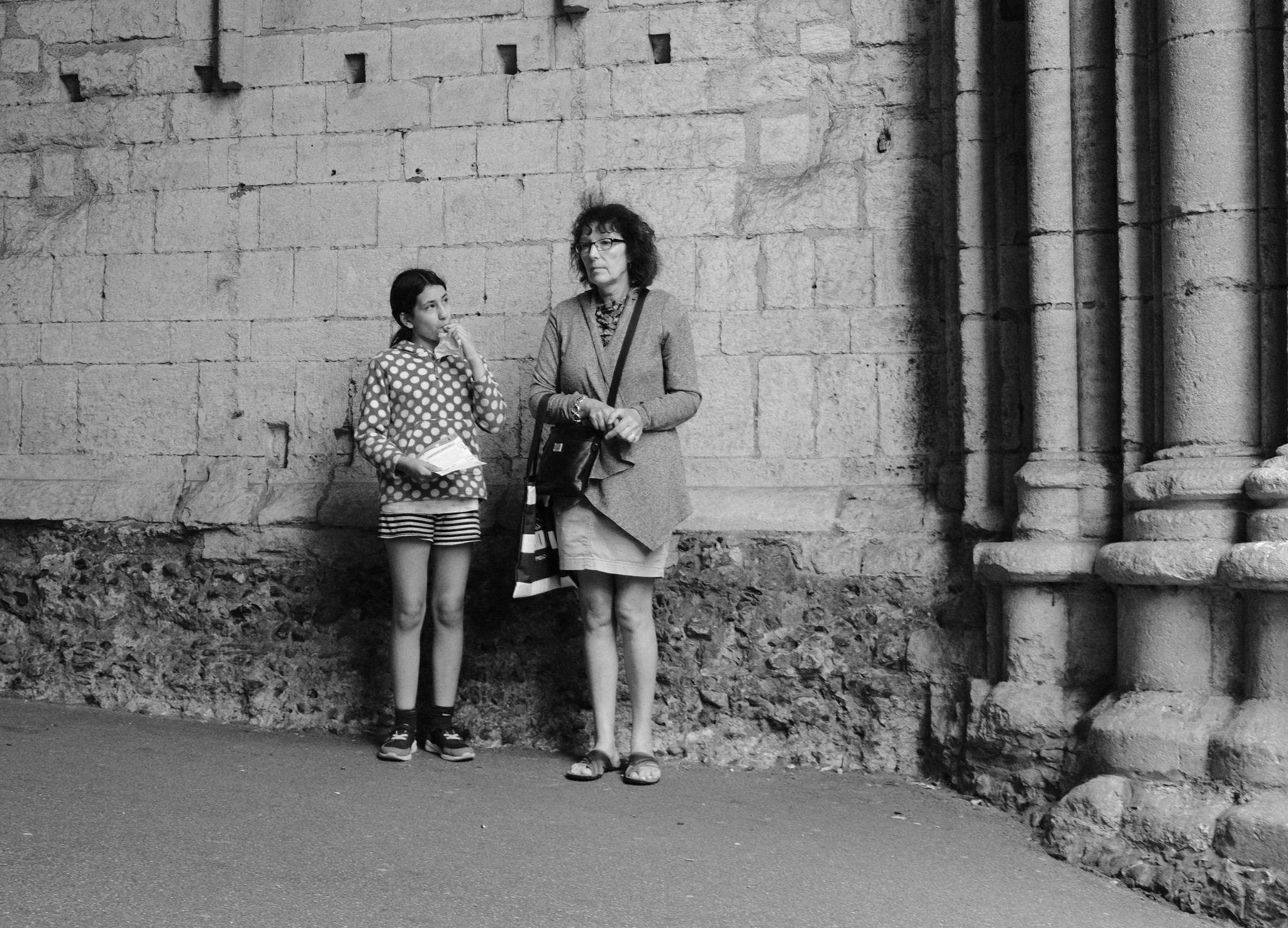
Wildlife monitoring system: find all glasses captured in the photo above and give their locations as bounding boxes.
[573,238,626,255]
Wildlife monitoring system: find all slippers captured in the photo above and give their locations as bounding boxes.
[567,749,625,780]
[624,752,660,784]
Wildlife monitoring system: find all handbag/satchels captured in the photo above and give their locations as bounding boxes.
[536,422,601,496]
[511,393,577,598]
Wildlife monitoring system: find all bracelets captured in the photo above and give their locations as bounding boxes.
[571,395,588,422]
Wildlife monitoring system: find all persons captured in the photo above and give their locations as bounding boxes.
[528,195,703,786]
[353,269,509,760]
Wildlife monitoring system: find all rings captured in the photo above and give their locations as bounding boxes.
[631,434,637,438]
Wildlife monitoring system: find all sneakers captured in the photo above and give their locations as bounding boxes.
[378,723,425,761]
[425,724,475,761]
